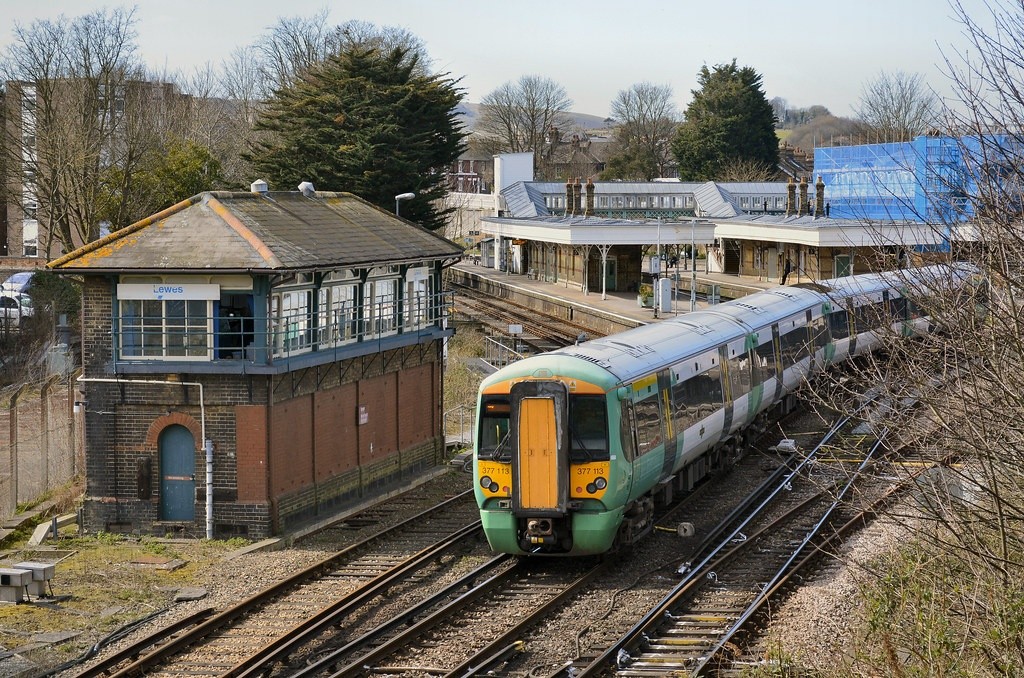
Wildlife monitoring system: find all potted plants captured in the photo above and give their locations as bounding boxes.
[637,284,654,308]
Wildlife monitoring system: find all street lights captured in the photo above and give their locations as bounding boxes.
[466,209,483,260]
[646,216,672,307]
[679,218,709,311]
[395,193,416,216]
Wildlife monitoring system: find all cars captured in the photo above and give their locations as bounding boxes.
[0,290,36,330]
[2,272,37,295]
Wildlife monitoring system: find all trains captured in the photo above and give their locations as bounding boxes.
[470,262,990,558]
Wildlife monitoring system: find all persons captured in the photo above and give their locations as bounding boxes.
[781,257,791,285]
[670,254,678,268]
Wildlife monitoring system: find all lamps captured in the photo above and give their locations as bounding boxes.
[74,401,88,413]
